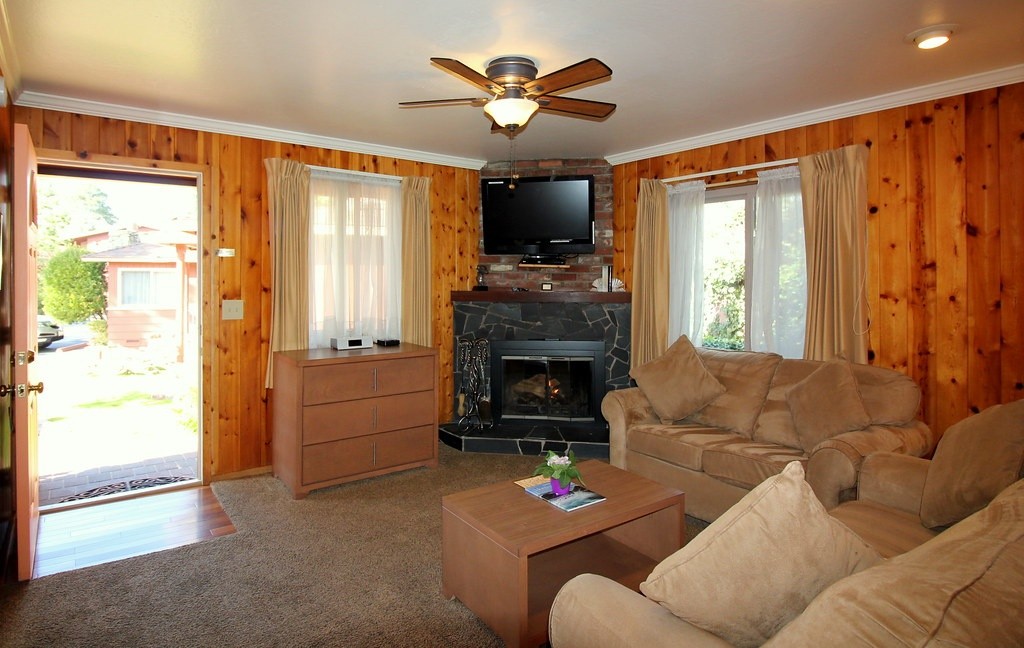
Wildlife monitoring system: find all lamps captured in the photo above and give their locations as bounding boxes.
[915,30,949,50]
[483,98,540,190]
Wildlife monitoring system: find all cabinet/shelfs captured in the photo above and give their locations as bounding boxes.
[274,342,440,499]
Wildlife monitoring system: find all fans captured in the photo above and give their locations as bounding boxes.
[399,57,617,129]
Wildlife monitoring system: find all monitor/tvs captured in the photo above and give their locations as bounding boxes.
[481,174,596,264]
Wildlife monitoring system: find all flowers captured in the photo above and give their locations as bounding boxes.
[531,450,585,489]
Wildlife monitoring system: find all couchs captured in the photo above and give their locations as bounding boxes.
[601,347,931,526]
[548,451,1024,648]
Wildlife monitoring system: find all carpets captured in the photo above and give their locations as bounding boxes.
[0,441,711,648]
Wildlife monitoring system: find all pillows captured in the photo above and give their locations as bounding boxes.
[786,350,870,453]
[629,334,727,425]
[920,399,1024,528]
[640,461,887,648]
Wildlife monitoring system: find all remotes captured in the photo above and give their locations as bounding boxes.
[512,287,529,292]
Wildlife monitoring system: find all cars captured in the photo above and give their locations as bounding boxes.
[36,315,64,348]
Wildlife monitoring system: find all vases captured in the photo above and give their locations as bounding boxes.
[551,477,570,496]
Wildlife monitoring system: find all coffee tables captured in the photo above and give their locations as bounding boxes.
[443,459,685,648]
